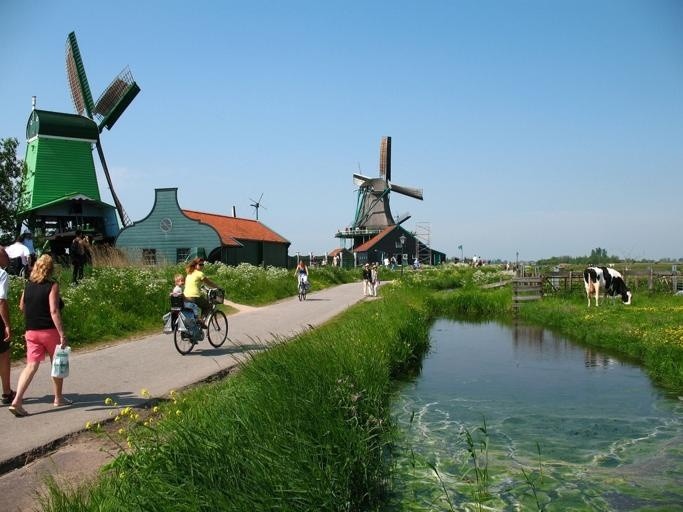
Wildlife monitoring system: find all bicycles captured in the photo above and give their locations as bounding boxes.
[295,274,306,301]
[171,285,229,357]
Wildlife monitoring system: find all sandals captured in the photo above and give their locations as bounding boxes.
[2,389,16,404]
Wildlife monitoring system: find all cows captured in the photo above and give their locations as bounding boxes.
[582,266,633,307]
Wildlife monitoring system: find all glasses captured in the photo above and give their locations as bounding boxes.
[199,264,204,266]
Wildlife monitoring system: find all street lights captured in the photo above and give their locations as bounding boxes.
[457,244,463,262]
[515,251,519,269]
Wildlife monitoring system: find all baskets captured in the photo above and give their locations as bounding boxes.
[207,290,225,303]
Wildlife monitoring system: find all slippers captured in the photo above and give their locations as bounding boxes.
[7,406,28,416]
[53,397,74,406]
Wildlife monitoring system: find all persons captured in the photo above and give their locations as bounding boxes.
[171,273,199,320]
[370,265,378,297]
[391,255,397,270]
[473,254,477,263]
[332,253,339,266]
[477,256,482,265]
[384,256,390,265]
[0,229,93,286]
[362,263,371,297]
[7,252,74,418]
[321,258,327,267]
[182,256,221,332]
[0,244,18,407]
[294,259,309,294]
